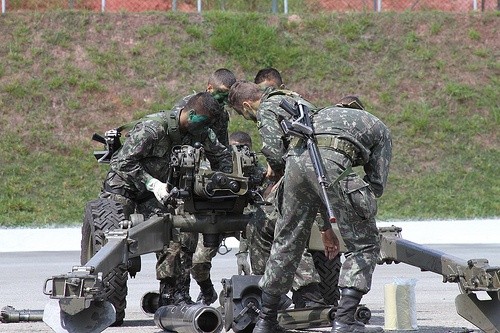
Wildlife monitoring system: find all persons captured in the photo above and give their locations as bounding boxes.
[254,68,305,309]
[156,68,236,306]
[190,130,268,307]
[99,92,233,307]
[252,95,392,333]
[228,79,328,326]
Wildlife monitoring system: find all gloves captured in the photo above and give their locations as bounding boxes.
[235,251,250,275]
[145,177,170,205]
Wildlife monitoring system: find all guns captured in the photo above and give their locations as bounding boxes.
[275,98,337,224]
[91,129,123,163]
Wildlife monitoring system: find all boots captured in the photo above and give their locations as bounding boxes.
[196,274,218,306]
[159,278,173,306]
[252,292,281,333]
[173,278,196,306]
[292,284,330,309]
[330,287,384,333]
[277,294,293,310]
